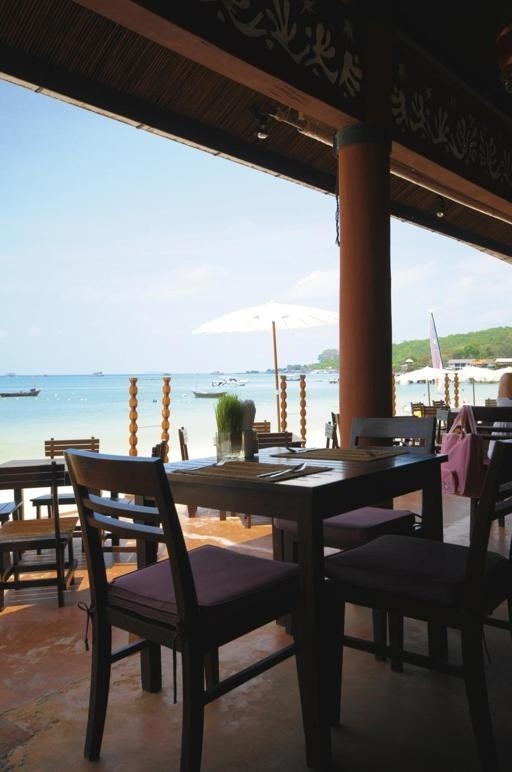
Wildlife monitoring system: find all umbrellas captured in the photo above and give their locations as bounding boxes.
[478,365,512,383]
[190,298,339,431]
[398,365,449,409]
[447,364,490,406]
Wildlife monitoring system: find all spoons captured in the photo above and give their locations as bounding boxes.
[266,461,307,479]
[285,445,316,453]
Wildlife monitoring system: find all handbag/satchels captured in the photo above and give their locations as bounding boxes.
[440,405,491,498]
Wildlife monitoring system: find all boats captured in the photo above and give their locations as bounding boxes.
[188,375,251,398]
[0,388,42,398]
[281,367,339,384]
[8,372,17,377]
[93,372,105,376]
